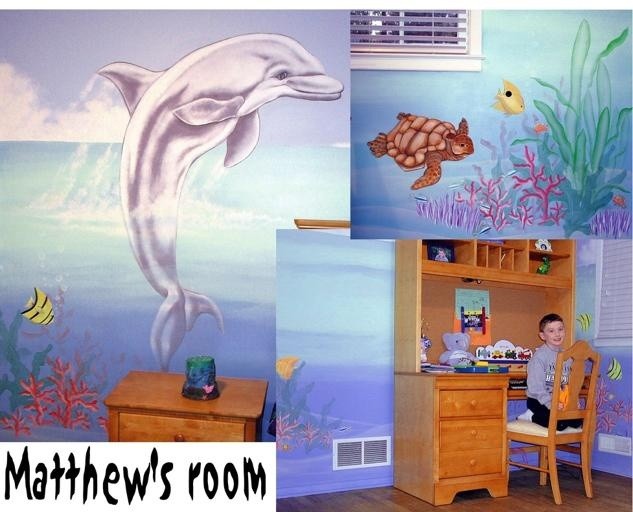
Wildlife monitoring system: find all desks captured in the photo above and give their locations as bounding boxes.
[395,233,575,503]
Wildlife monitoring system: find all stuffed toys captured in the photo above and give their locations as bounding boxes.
[439,331,480,367]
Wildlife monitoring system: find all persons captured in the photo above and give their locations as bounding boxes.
[516,313,583,430]
[435,248,449,262]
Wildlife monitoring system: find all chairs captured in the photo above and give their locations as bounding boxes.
[506,340,600,505]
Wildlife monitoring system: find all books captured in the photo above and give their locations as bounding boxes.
[423,363,456,374]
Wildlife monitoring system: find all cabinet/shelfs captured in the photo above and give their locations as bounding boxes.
[104,371,269,441]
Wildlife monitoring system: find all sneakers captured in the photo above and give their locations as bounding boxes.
[515,409,534,422]
